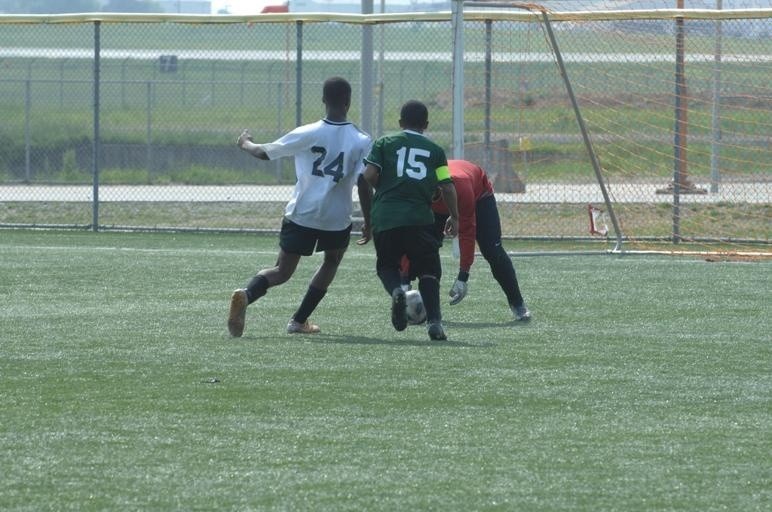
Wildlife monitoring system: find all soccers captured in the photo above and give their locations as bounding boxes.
[402,290,427,326]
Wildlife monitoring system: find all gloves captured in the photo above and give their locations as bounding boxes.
[449,270,470,307]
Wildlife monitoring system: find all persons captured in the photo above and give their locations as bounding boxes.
[397,158,533,322]
[227,74,375,339]
[363,99,459,342]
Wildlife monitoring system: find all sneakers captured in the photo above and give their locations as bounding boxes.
[228,288,248,338]
[427,318,448,341]
[391,287,408,331]
[510,301,530,321]
[287,318,321,335]
